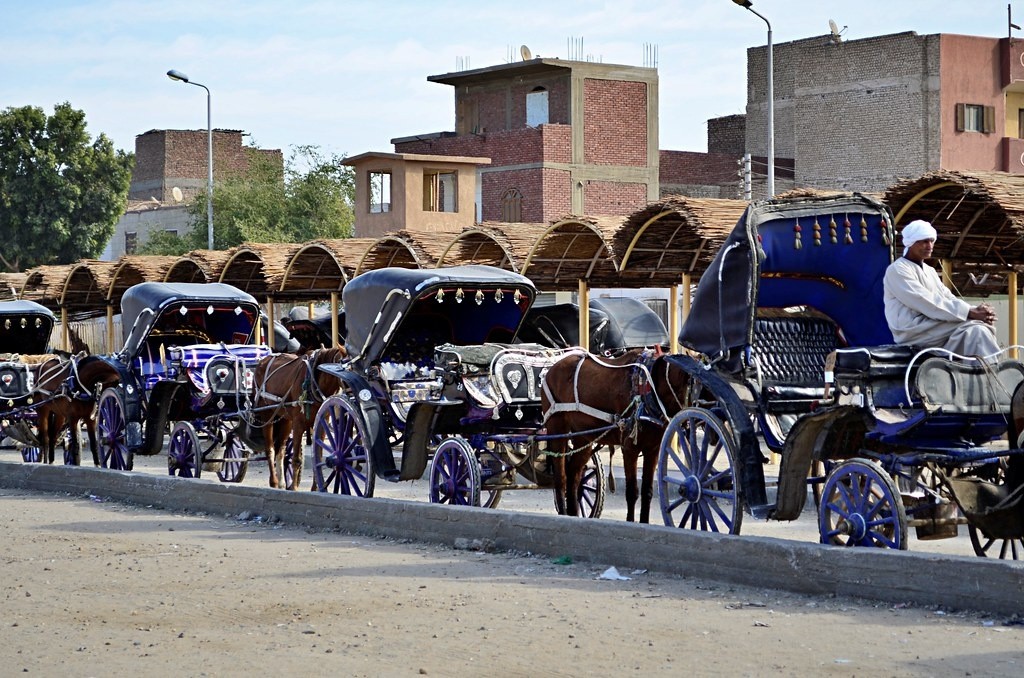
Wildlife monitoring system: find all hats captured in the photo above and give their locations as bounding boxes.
[902,220,937,257]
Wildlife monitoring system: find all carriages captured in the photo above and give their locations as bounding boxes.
[300,265,731,528]
[653,191,1024,564]
[0,299,129,467]
[88,282,361,491]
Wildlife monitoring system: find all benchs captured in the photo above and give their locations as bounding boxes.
[740,306,852,399]
[378,332,480,382]
[169,343,271,367]
[834,343,922,379]
[432,342,560,377]
[131,327,212,390]
[0,353,61,369]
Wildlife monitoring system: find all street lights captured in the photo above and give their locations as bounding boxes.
[732,0,776,205]
[168,69,214,251]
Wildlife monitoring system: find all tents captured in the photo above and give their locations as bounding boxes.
[0,170,1024,360]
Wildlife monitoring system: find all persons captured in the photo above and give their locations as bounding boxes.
[883,219,1002,363]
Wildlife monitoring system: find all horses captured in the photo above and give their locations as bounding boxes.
[540,348,727,524]
[251,342,348,492]
[32,355,121,468]
